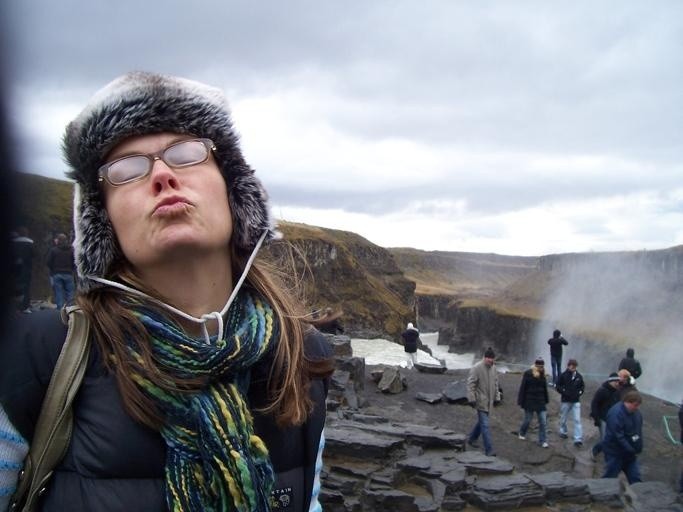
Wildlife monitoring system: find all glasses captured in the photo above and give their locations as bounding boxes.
[93,136,220,188]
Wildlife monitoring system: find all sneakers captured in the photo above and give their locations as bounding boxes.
[467,439,480,449]
[518,432,527,440]
[486,451,496,458]
[575,440,584,447]
[591,448,597,462]
[542,442,548,448]
[559,433,568,439]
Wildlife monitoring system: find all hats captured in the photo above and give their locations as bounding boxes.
[568,359,577,365]
[406,322,415,329]
[628,348,634,358]
[554,330,561,337]
[60,70,275,299]
[484,347,495,358]
[610,371,620,382]
[536,357,545,366]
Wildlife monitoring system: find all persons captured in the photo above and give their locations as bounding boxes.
[602,390,644,483]
[1,69,333,512]
[314,301,345,334]
[466,347,503,457]
[618,369,640,400]
[591,374,615,461]
[516,358,552,448]
[399,321,422,368]
[7,225,76,314]
[616,347,642,378]
[555,359,586,447]
[547,330,568,386]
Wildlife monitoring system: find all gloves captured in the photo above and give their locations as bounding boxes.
[494,400,499,407]
[470,402,477,408]
